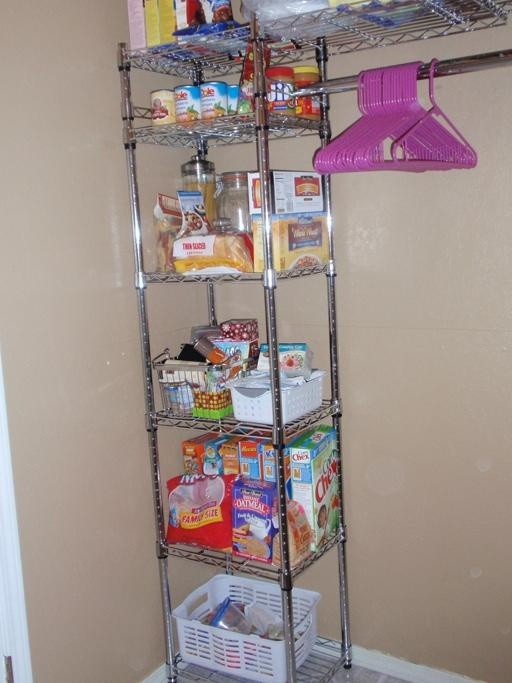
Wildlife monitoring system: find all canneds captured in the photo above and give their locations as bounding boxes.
[150,82,241,125]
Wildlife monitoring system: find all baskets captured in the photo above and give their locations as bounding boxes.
[171,574,322,683]
[152,348,259,420]
[225,369,327,425]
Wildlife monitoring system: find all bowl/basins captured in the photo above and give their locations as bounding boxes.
[202,596,253,644]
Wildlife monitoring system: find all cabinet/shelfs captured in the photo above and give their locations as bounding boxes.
[117,0,512,683]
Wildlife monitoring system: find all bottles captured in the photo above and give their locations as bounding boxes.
[212,218,236,234]
[207,364,223,377]
[181,155,217,222]
[263,66,296,119]
[192,336,228,364]
[216,170,250,234]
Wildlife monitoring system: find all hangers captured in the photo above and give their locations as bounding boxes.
[313,58,479,174]
